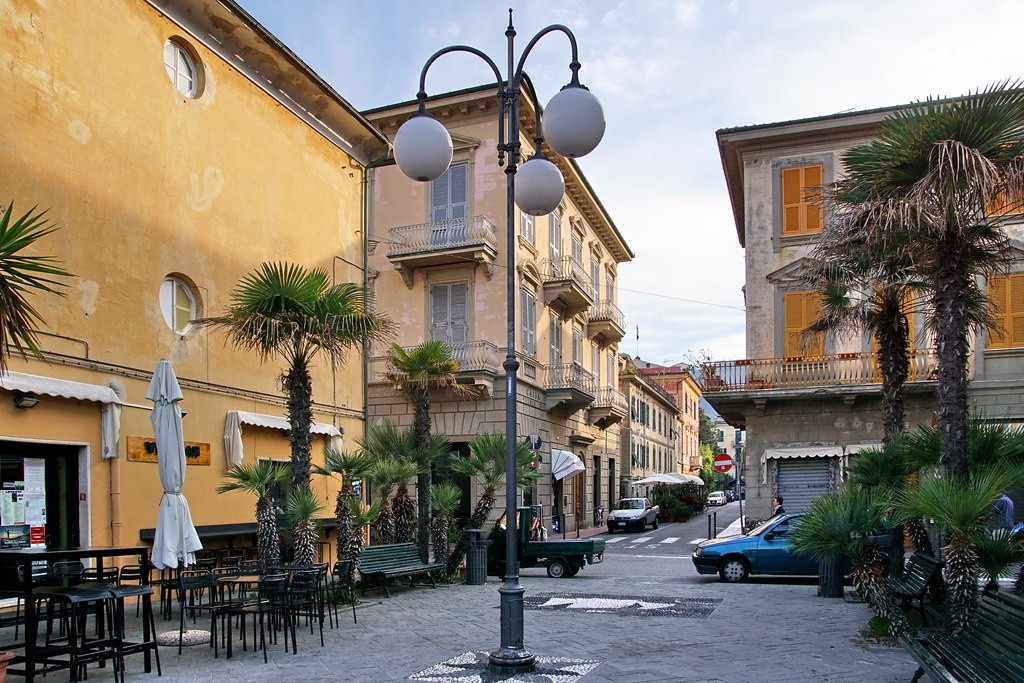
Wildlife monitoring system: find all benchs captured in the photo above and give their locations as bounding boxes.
[896,588,1024,683]
[877,551,951,633]
[356,542,444,599]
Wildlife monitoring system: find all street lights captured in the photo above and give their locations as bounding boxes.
[393,8,606,674]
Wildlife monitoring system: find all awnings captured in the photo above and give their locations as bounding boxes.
[551,449,585,481]
[666,473,704,485]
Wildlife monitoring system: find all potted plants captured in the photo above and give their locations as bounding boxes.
[683,347,724,389]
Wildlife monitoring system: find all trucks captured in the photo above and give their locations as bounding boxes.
[486,508,605,582]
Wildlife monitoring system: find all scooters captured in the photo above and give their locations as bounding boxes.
[968,515,1024,557]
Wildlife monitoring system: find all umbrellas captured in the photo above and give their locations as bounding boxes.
[630,474,685,488]
[145,358,204,571]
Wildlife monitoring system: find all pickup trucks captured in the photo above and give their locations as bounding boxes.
[607,498,660,534]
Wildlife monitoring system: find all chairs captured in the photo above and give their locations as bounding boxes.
[317,560,357,628]
[52,562,100,635]
[72,566,119,637]
[178,572,243,660]
[167,558,217,623]
[238,560,264,573]
[14,564,65,642]
[227,572,296,664]
[209,556,246,614]
[211,567,262,652]
[287,562,329,630]
[119,563,147,618]
[136,556,175,618]
[264,556,289,572]
[266,569,323,653]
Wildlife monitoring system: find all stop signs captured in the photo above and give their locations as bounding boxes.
[713,453,732,472]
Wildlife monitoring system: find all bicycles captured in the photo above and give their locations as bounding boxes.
[594,505,604,528]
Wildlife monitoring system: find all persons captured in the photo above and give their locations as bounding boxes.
[984,488,1014,531]
[767,497,789,535]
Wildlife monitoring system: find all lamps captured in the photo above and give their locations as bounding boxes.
[14,394,40,408]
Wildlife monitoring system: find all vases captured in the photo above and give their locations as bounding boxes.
[0,651,16,683]
[748,379,764,389]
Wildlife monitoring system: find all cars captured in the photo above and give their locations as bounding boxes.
[692,512,893,583]
[707,492,727,506]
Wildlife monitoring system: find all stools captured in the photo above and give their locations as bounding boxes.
[33,586,119,683]
[76,581,161,683]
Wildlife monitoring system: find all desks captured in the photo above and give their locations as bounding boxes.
[0,546,151,683]
[218,576,296,654]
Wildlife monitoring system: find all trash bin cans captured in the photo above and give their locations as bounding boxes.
[460,529,493,585]
